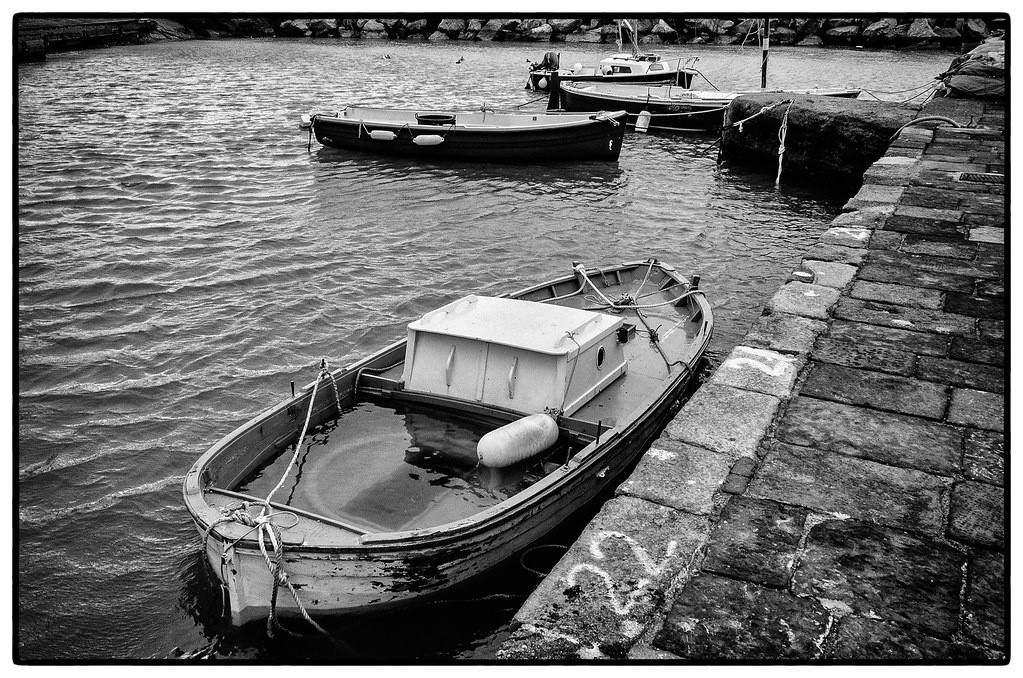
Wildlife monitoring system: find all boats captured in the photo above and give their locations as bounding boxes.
[735,86,861,102]
[306,100,631,169]
[526,48,699,93]
[559,79,744,131]
[179,254,714,648]
